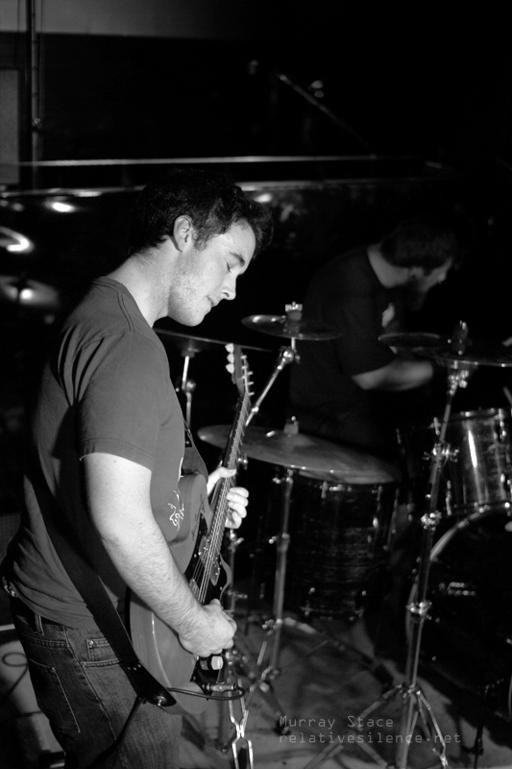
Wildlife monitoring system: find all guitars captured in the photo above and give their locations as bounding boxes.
[129,344,250,718]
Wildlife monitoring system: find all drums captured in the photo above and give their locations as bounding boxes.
[405,508,510,719]
[396,408,510,515]
[377,331,472,426]
[222,443,401,622]
[431,353,512,410]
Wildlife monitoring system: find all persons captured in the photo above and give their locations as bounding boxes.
[1,170,276,769]
[287,202,455,659]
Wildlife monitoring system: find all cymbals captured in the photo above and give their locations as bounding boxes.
[243,314,344,342]
[196,422,375,476]
[153,328,273,352]
